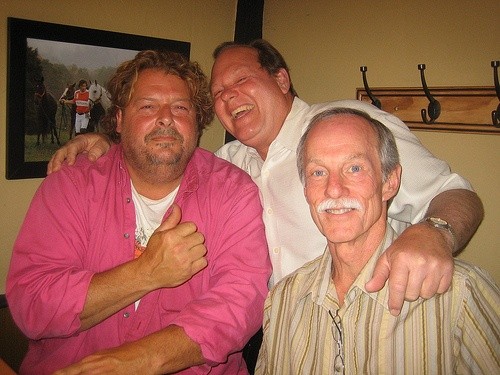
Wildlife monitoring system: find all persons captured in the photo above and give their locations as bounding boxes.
[62,79,91,135]
[254,107,500,375]
[47,40,485,375]
[5,45,270,375]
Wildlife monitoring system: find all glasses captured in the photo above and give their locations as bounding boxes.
[329,309,344,374]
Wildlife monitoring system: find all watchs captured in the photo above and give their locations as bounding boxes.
[418,217,457,256]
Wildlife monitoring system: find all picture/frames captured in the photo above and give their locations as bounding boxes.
[6,15,192,180]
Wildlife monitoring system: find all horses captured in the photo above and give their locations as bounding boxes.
[31,76,112,144]
